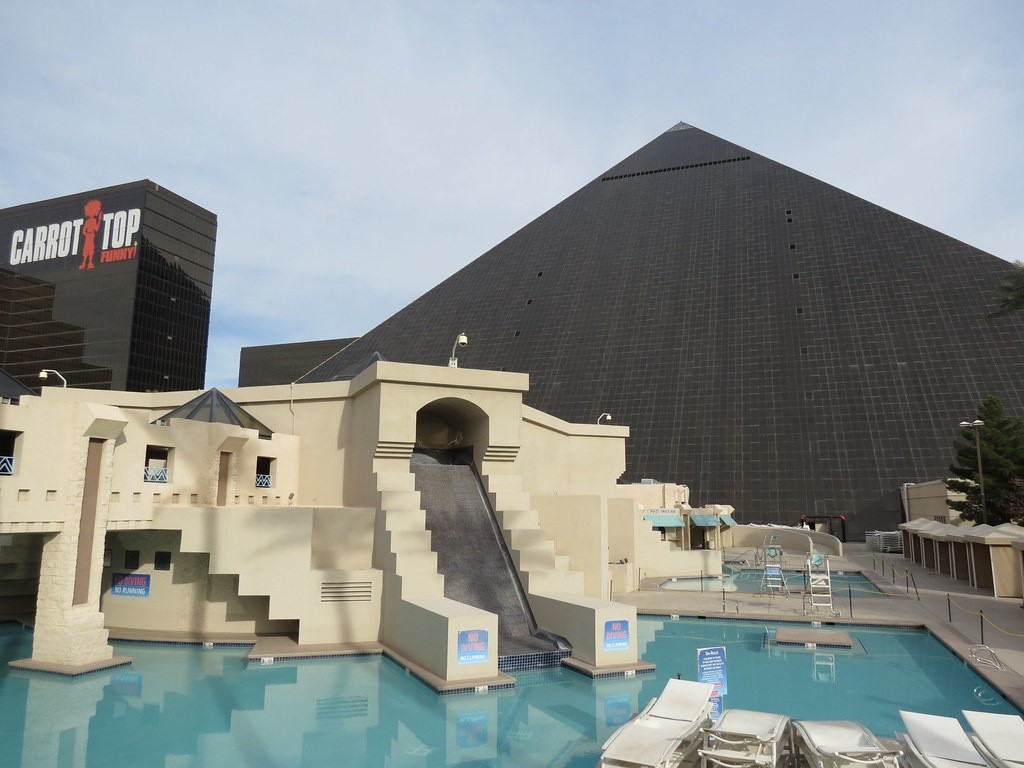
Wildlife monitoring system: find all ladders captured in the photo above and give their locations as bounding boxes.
[811,652,837,685]
[801,551,841,616]
[759,565,791,597]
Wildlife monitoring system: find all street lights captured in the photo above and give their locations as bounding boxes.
[959,419,987,524]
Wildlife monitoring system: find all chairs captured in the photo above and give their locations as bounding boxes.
[806,554,841,618]
[601,678,1024,768]
[764,545,790,595]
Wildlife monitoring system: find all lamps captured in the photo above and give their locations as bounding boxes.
[598,413,611,424]
[39,369,67,388]
[449,333,468,369]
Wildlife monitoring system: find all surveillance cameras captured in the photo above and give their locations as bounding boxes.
[606,416,611,422]
[39,371,48,381]
[458,336,468,347]
